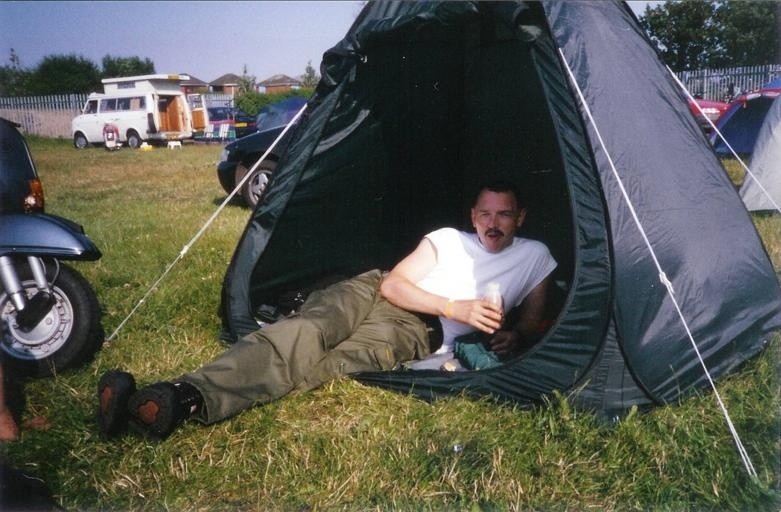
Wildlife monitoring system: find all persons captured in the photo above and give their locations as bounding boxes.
[0,367,48,446]
[97,163,562,449]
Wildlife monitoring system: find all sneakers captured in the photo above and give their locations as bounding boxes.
[129,382,182,441]
[96,370,137,436]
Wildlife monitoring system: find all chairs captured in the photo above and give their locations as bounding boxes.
[200,123,230,145]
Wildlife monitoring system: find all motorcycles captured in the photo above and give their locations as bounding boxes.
[0,118,102,378]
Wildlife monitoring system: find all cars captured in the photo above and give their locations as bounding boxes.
[208,107,257,138]
[218,125,286,209]
[686,92,732,136]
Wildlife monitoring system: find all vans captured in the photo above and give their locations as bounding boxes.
[71,90,209,148]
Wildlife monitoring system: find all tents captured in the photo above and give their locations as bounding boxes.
[214,1,780,422]
[702,73,781,214]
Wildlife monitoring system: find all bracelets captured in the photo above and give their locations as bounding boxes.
[442,298,459,318]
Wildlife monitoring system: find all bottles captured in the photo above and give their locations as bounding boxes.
[482,282,504,311]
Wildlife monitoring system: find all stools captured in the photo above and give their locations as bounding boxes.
[167,140,182,151]
